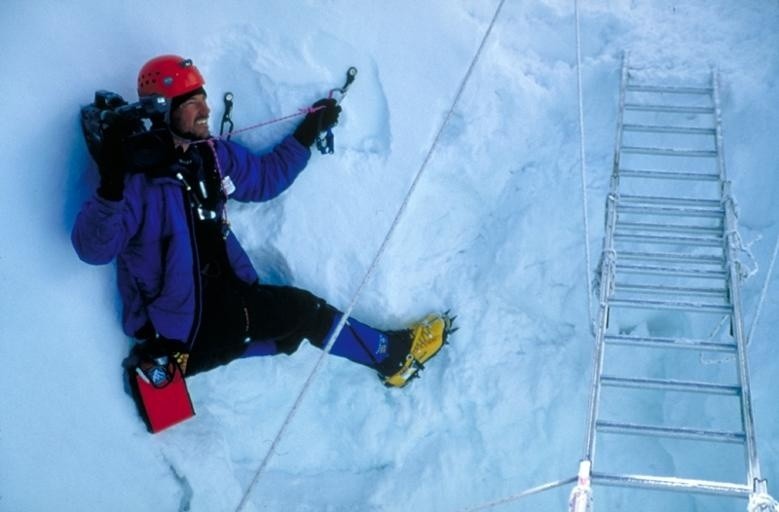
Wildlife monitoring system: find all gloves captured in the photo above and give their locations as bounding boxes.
[88,142,132,202]
[292,98,343,148]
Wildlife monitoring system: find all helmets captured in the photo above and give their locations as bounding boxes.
[136,54,207,100]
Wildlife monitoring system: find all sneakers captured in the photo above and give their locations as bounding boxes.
[375,308,458,391]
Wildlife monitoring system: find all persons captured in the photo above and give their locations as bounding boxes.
[68,52,450,391]
